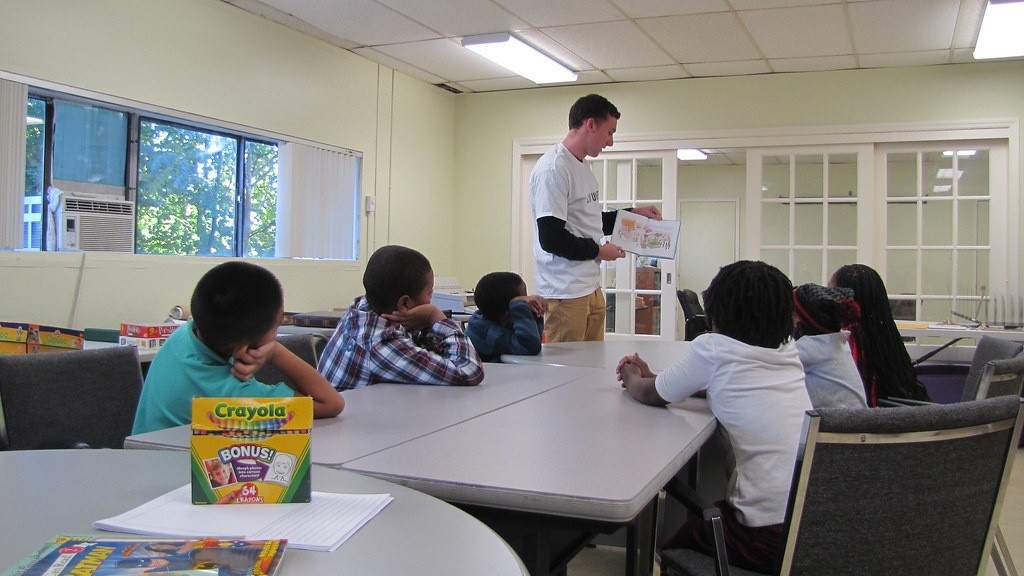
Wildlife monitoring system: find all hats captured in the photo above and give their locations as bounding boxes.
[794,283,861,334]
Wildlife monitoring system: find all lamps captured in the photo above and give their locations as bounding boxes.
[973,0,1024,60]
[677,149,707,161]
[461,33,578,84]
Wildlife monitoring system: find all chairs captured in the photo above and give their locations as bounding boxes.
[660,394,1024,576]
[256,332,318,395]
[677,289,713,341]
[85,328,121,343]
[876,349,1024,576]
[959,335,1024,404]
[0,345,144,449]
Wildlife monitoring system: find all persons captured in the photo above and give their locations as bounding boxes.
[129,257,345,437]
[616,259,815,576]
[828,262,930,409]
[464,271,549,363]
[317,244,485,395]
[209,459,236,489]
[788,282,870,411]
[530,92,661,344]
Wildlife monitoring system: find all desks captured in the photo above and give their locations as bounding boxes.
[500,338,694,374]
[341,367,720,576]
[124,363,603,471]
[0,449,530,576]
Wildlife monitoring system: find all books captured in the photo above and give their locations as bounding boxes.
[278,312,305,326]
[609,207,682,261]
[0,533,289,576]
[293,311,349,328]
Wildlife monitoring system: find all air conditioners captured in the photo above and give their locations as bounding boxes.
[53,190,136,252]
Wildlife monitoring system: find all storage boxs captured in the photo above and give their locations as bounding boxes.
[121,322,181,338]
[119,336,168,350]
[0,321,85,349]
[0,341,81,355]
